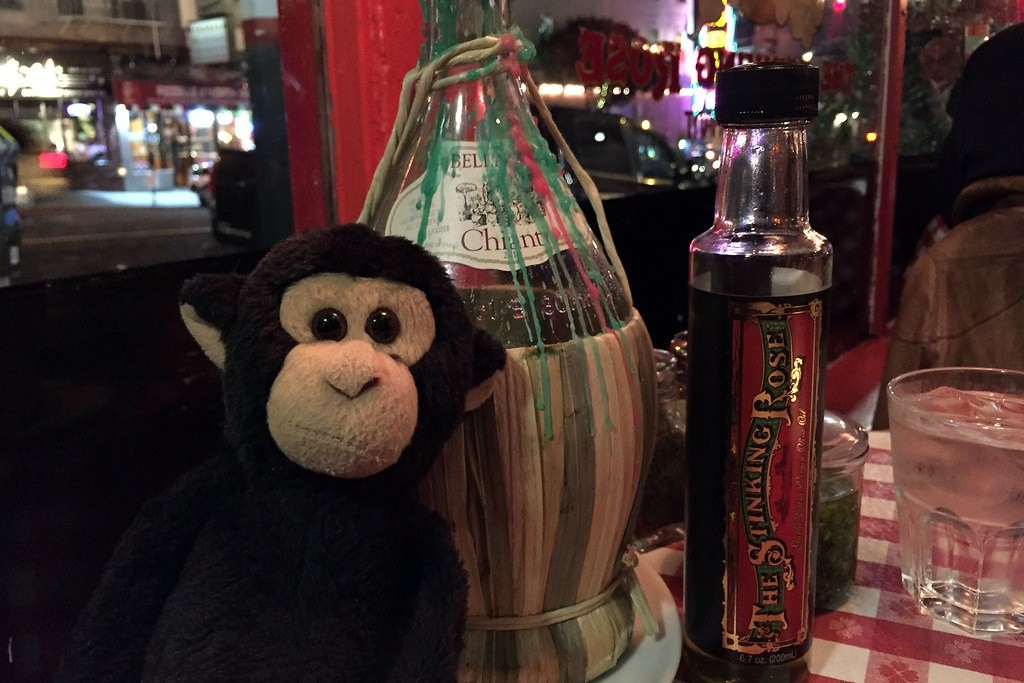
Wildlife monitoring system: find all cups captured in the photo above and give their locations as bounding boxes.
[886,365,1024,637]
[812,409,871,617]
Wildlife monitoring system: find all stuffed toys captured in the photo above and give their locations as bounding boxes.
[57,220,507,682]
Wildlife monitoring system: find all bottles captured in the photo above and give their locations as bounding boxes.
[638,347,690,540]
[366,1,632,348]
[681,59,832,683]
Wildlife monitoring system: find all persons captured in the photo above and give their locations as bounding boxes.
[873,20,1023,431]
